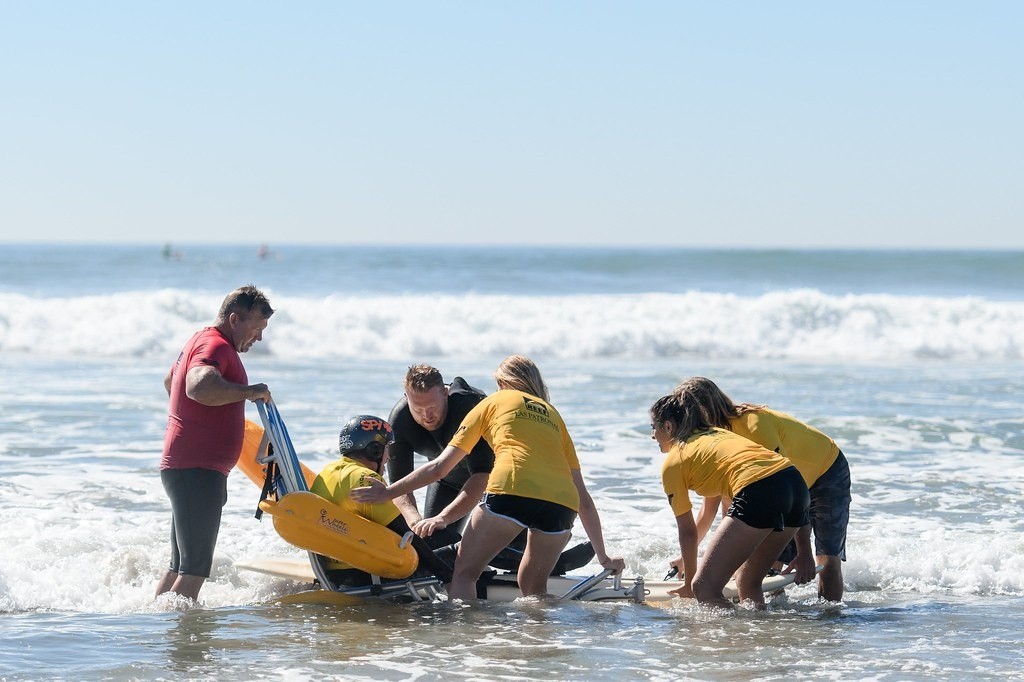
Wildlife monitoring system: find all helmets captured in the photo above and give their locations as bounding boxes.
[339,416,395,454]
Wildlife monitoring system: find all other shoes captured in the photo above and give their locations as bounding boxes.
[550,541,595,576]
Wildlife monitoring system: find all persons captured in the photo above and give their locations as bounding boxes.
[309,353,626,604]
[649,376,852,606]
[154,281,275,603]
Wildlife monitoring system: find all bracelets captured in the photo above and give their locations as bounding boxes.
[601,557,610,565]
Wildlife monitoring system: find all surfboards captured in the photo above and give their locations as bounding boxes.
[234,557,826,607]
